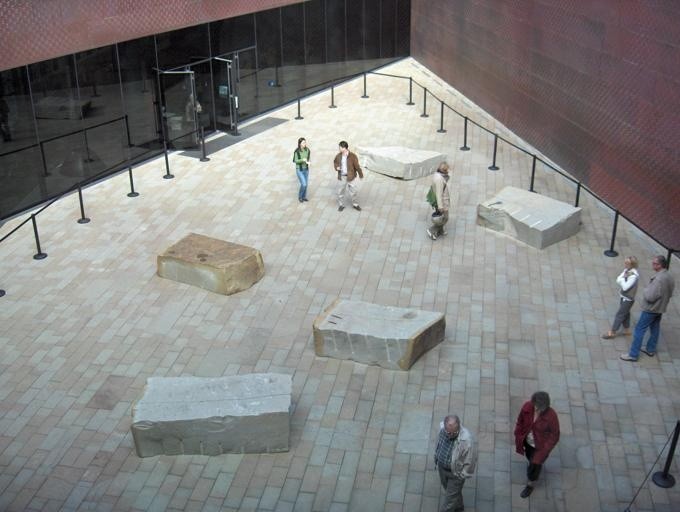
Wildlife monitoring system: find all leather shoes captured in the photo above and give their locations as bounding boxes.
[621,353,638,361]
[299,197,303,202]
[339,205,345,211]
[353,204,361,210]
[640,347,654,356]
[303,198,307,201]
[521,485,534,498]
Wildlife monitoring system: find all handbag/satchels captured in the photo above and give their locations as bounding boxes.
[426,189,437,208]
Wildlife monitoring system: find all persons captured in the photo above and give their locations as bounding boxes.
[513,391,560,499]
[433,413,479,512]
[293,137,312,203]
[619,255,676,362]
[600,254,640,340]
[427,160,451,242]
[0,91,12,143]
[334,140,364,213]
[184,92,202,148]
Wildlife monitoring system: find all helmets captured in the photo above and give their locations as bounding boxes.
[432,211,443,224]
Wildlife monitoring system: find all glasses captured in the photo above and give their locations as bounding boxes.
[445,430,459,434]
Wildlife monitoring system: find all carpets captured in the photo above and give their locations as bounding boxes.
[177,116,289,161]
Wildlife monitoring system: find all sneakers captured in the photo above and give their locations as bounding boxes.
[427,229,436,240]
[602,331,616,339]
[439,230,448,235]
[618,329,632,336]
